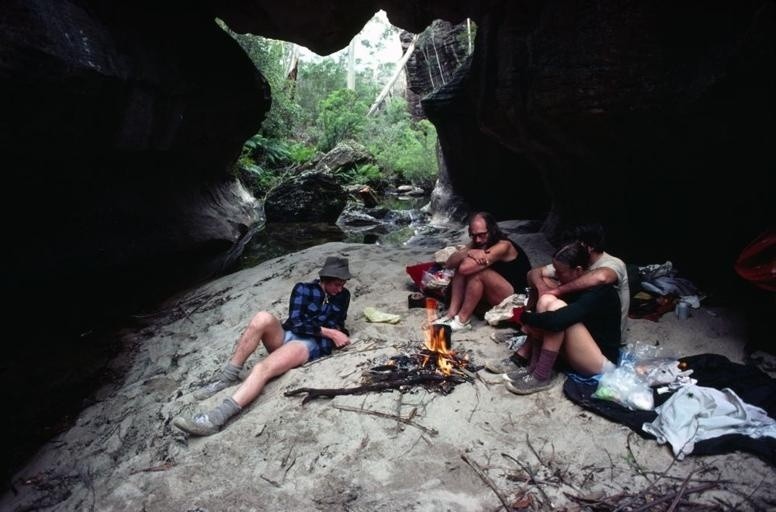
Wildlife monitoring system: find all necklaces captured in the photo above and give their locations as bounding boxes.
[322,293,330,306]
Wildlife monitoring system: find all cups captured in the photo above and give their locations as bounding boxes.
[675,301,691,319]
[430,323,452,351]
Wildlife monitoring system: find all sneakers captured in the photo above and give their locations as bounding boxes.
[193,380,238,400]
[174,416,218,436]
[486,356,555,395]
[434,315,471,333]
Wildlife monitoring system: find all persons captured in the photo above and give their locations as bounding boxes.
[173,257,351,436]
[503,243,622,395]
[433,210,532,334]
[483,227,630,374]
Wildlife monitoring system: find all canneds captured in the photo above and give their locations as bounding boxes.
[679,301,689,321]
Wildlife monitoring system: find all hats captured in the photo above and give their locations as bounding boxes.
[318,257,351,280]
